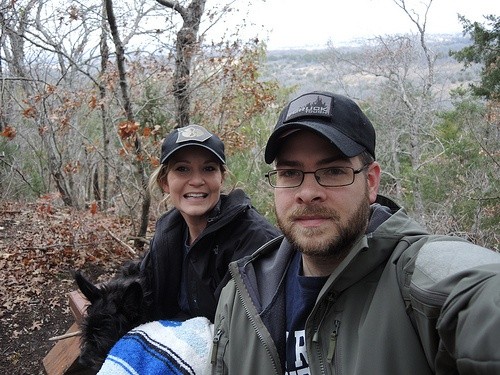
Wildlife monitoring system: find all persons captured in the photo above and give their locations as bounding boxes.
[113,125,288,375]
[210,91,500,375]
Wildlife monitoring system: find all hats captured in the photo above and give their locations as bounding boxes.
[265,90,376,165]
[160,124,226,164]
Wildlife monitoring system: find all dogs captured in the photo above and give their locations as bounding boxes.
[70,248,158,375]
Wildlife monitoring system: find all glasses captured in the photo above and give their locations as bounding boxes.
[265,163,368,188]
